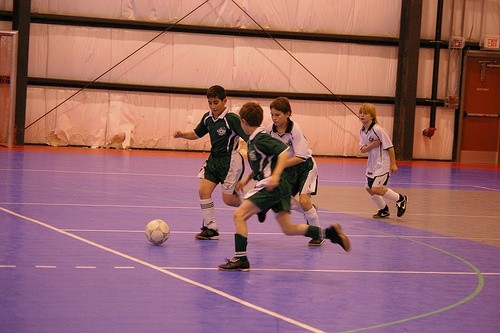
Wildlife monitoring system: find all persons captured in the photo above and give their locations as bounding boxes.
[218,102,352,272]
[266,97,327,246]
[358,103,408,219]
[172,85,266,240]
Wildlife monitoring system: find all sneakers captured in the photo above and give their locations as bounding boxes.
[396,194,408,217]
[373,205,390,218]
[258,211,266,223]
[195,226,219,240]
[329,223,350,252]
[219,256,250,272]
[307,204,317,225]
[309,238,325,245]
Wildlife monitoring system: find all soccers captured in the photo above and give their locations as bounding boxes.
[145,219,170,244]
